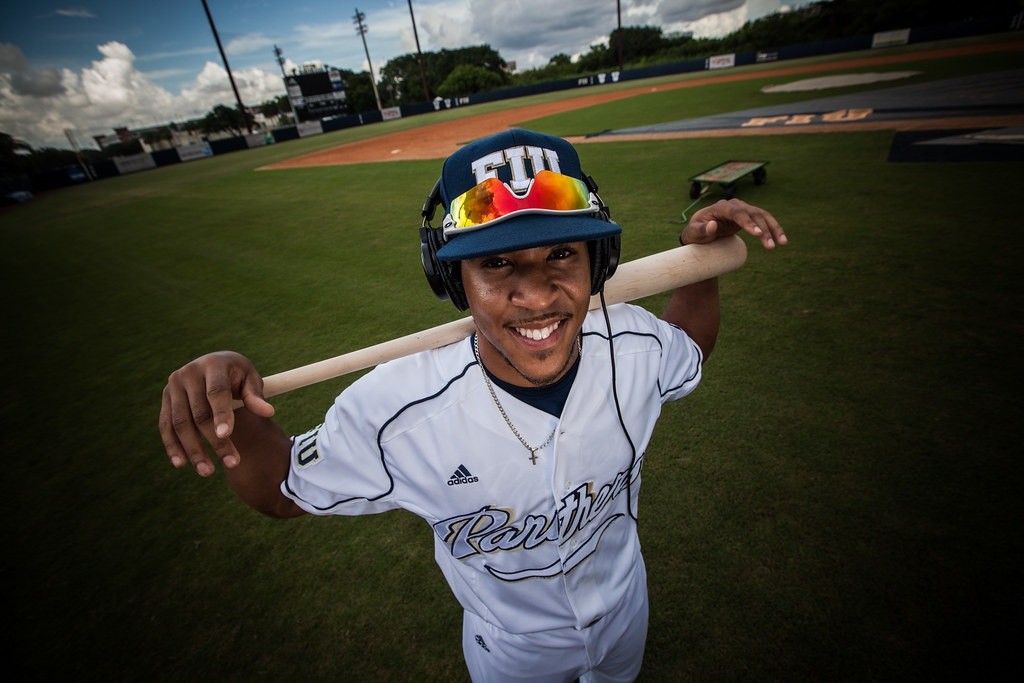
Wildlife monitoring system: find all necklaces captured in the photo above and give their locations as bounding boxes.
[475,332,582,466]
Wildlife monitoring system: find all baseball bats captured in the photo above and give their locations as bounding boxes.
[227,235,749,409]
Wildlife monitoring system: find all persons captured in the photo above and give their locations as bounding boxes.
[159,130,787,680]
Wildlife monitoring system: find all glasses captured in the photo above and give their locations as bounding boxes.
[443,170,598,240]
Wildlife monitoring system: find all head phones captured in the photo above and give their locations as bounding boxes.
[419,169,619,312]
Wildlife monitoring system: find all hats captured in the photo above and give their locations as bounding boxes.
[437,129,622,261]
